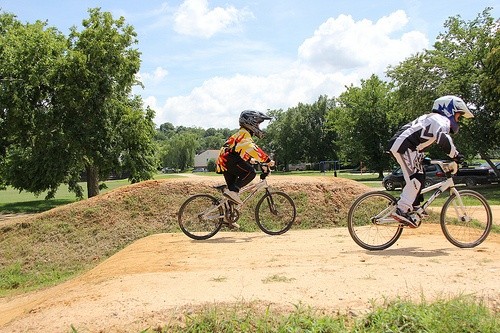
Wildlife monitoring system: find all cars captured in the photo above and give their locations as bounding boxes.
[282,161,339,172]
[192,168,209,173]
[382,161,500,191]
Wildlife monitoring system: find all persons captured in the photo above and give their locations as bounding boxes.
[386,95,474,228]
[215,110,276,228]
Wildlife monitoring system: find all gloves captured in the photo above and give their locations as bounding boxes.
[454,154,465,164]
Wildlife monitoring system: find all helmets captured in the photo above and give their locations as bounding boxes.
[431,95,474,135]
[239,110,272,140]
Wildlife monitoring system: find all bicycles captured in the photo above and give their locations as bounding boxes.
[348,157,492,250]
[178,161,296,240]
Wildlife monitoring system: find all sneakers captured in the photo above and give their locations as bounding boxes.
[413,206,429,217]
[232,222,240,228]
[393,208,418,228]
[223,189,243,205]
